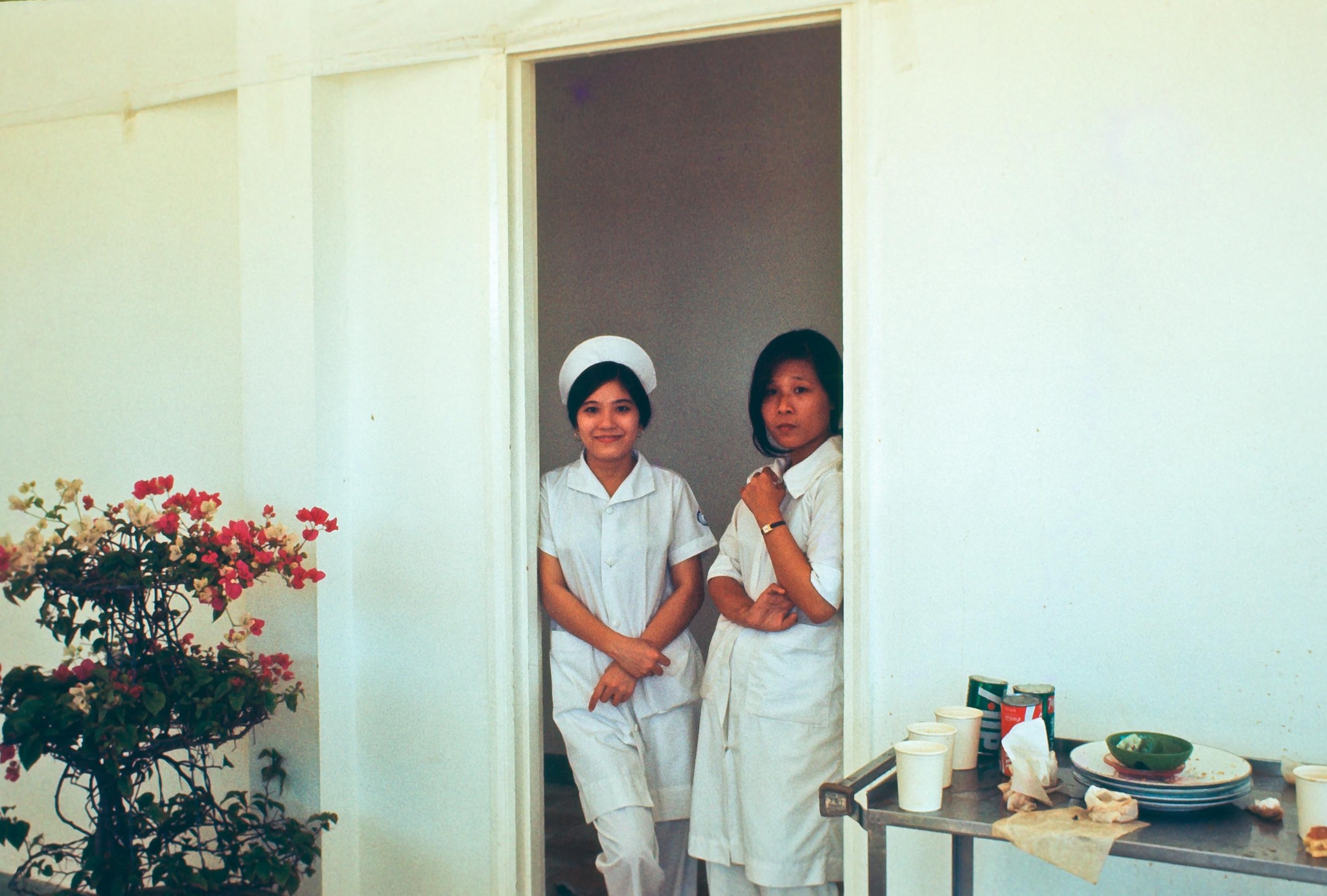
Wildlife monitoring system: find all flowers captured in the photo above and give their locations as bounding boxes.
[0,475,339,896]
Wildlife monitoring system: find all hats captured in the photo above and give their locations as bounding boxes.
[558,336,658,404]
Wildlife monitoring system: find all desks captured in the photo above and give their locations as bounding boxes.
[819,738,1327,896]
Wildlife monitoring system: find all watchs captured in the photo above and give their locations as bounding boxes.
[761,520,788,535]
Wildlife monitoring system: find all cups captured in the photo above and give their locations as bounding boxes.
[906,721,958,789]
[894,740,949,813]
[1293,765,1327,840]
[934,705,983,770]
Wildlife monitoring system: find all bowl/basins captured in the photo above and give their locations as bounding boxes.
[1105,731,1194,771]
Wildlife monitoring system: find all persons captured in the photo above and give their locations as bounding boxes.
[537,335,717,896]
[687,329,844,896]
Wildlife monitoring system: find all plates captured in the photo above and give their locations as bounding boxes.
[1070,740,1254,811]
[1103,753,1185,782]
